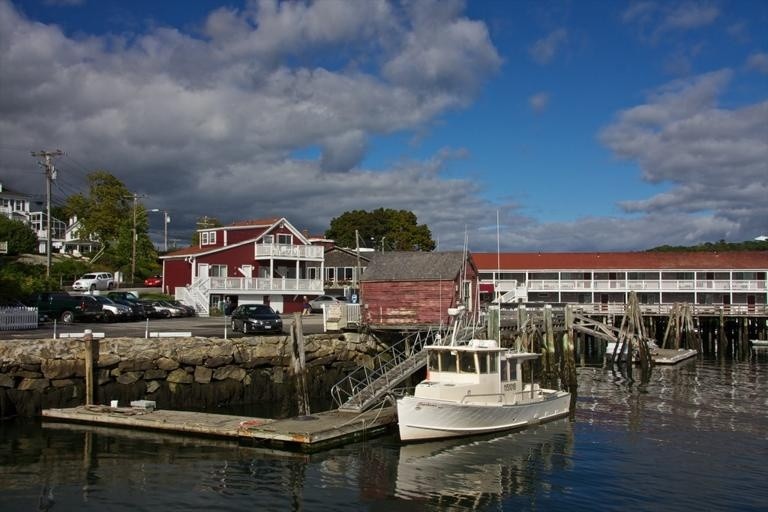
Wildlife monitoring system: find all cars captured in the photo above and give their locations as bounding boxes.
[36,290,195,324]
[231,304,282,334]
[308,294,351,313]
[144,275,162,287]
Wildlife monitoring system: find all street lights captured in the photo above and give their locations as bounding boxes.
[131,209,160,287]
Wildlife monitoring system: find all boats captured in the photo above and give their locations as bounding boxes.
[749,339,768,345]
[395,210,571,442]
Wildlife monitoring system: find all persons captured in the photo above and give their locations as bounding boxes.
[300,300,312,316]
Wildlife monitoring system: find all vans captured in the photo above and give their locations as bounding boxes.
[72,272,114,291]
